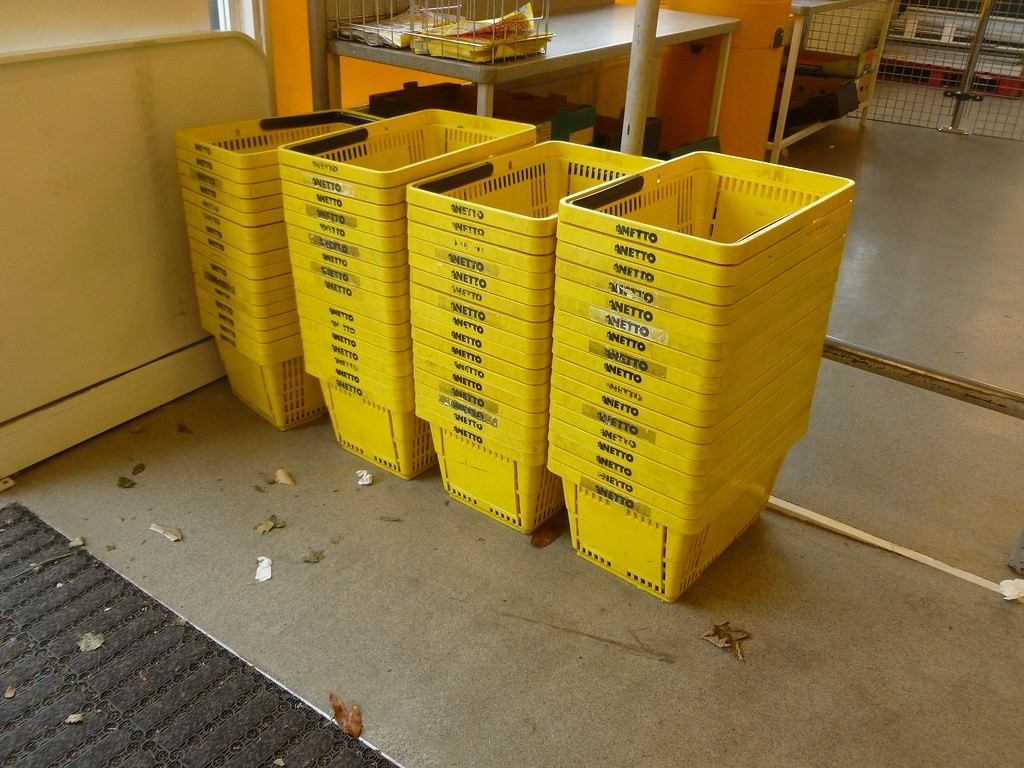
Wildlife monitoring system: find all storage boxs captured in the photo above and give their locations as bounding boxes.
[168,98,863,594]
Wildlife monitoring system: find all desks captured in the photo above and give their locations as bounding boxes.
[331,2,742,160]
